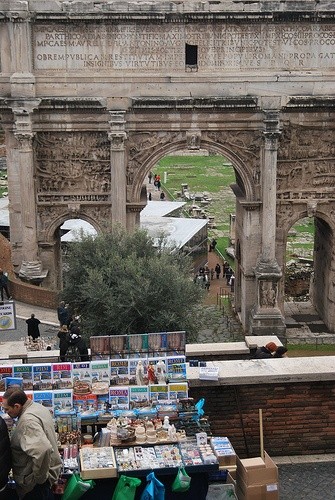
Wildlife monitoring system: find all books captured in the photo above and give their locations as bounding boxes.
[0,354,189,419]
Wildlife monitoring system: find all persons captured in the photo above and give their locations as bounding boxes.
[147,172,165,200]
[26,313,40,343]
[0,268,13,301]
[192,261,235,291]
[56,301,90,363]
[209,238,217,252]
[254,342,288,359]
[0,384,63,500]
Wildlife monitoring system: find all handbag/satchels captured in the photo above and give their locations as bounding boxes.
[171,467,191,492]
[62,473,96,499]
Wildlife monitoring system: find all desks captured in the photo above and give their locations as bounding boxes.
[82,422,110,433]
[0,441,219,500]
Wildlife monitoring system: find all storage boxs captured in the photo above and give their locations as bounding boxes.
[207,438,280,500]
[79,446,219,480]
